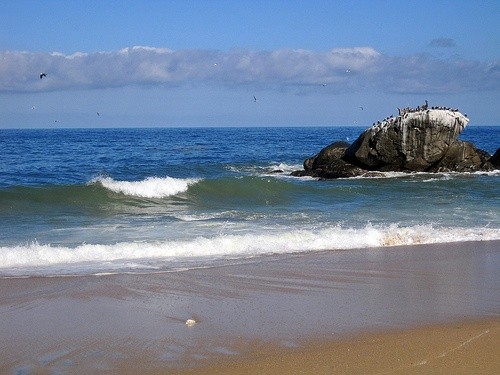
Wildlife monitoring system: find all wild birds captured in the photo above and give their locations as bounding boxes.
[32,105,37,110]
[253,95,257,102]
[346,69,351,73]
[360,106,364,111]
[321,84,326,86]
[97,111,100,116]
[39,73,47,79]
